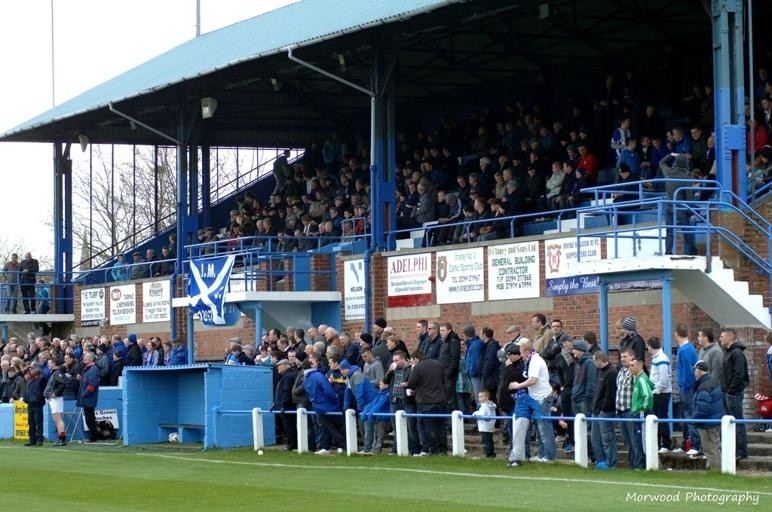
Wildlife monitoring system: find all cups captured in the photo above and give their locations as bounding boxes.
[684,437,692,452]
[406,389,412,396]
[390,363,397,370]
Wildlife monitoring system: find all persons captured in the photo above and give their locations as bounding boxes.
[111,67,772,251]
[1,250,772,476]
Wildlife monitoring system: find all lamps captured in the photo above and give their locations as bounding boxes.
[337,53,349,72]
[200,97,217,120]
[269,77,281,92]
[77,134,90,152]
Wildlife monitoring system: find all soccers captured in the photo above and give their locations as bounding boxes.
[168,433,178,444]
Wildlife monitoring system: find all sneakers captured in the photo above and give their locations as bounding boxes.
[24,441,35,446]
[37,440,43,446]
[658,447,707,460]
[313,447,431,457]
[58,439,66,446]
[507,436,574,467]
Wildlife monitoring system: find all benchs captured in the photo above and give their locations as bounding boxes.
[522,167,668,233]
[160,423,204,444]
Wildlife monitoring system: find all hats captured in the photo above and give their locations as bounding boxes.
[29,361,40,368]
[276,359,290,365]
[694,361,709,372]
[559,316,636,352]
[463,326,475,337]
[128,334,136,343]
[98,343,106,352]
[172,339,182,343]
[506,326,520,333]
[339,318,387,368]
[229,337,241,344]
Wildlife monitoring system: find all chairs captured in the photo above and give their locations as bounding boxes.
[63,402,85,442]
